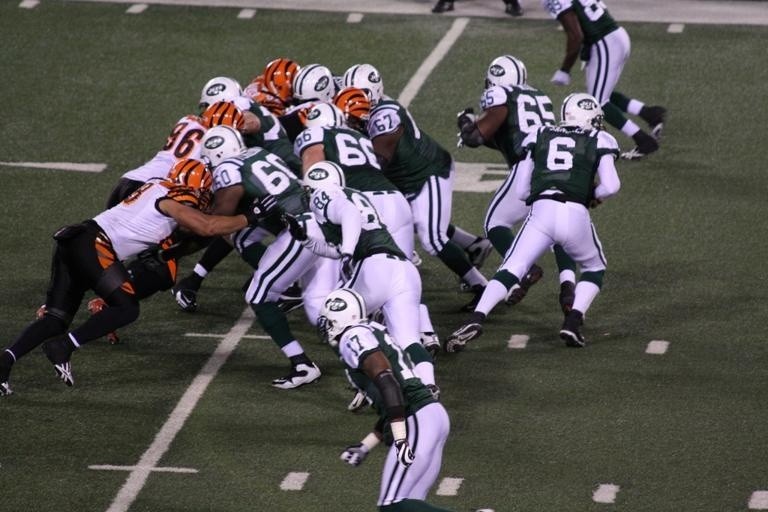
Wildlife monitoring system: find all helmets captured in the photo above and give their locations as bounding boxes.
[168,57,384,209]
[485,55,528,88]
[317,289,365,343]
[560,92,605,128]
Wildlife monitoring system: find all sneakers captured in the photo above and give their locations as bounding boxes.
[622,139,659,159]
[433,0,453,11]
[506,2,523,16]
[649,107,666,138]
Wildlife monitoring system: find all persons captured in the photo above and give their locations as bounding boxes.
[544,0,668,162]
[455,53,576,317]
[429,1,524,17]
[440,89,623,355]
[2,48,494,414]
[315,287,452,511]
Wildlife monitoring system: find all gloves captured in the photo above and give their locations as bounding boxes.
[457,107,475,130]
[339,441,367,465]
[551,70,571,85]
[244,196,277,223]
[395,438,415,467]
[282,213,305,241]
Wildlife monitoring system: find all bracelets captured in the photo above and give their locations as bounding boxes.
[390,421,406,445]
[361,432,380,449]
